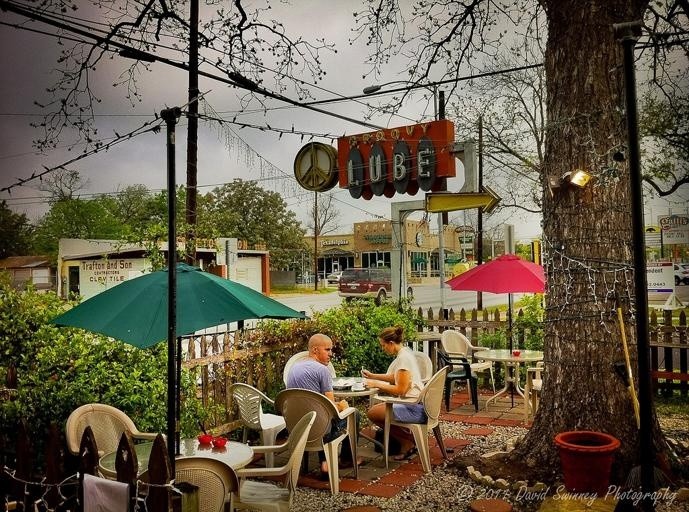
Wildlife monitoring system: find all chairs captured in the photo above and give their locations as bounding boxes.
[234,410,316,512]
[138,457,239,512]
[383,365,450,473]
[228,383,286,468]
[273,388,359,494]
[64,402,159,457]
[523,361,544,419]
[432,346,479,412]
[368,351,433,408]
[440,329,497,405]
[281,350,337,388]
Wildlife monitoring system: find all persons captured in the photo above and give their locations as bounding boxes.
[287,333,367,482]
[359,324,427,462]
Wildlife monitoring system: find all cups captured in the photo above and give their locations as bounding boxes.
[351,385,366,391]
[353,381,366,389]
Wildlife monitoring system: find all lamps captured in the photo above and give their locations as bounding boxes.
[548,168,592,189]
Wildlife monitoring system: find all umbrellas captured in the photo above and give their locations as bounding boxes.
[442,252,548,409]
[45,260,312,456]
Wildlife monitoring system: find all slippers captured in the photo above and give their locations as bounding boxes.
[337,454,366,469]
[394,447,416,461]
[317,470,328,481]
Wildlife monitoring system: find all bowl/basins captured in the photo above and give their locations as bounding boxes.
[513,351,521,356]
[197,435,228,448]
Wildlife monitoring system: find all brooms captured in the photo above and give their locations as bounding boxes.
[617,307,678,491]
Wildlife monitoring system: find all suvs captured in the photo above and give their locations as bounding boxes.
[673,262,688,287]
[337,266,414,306]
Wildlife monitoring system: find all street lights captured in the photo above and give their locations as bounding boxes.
[361,79,447,311]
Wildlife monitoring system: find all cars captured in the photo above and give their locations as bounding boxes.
[325,270,343,285]
[296,272,321,284]
